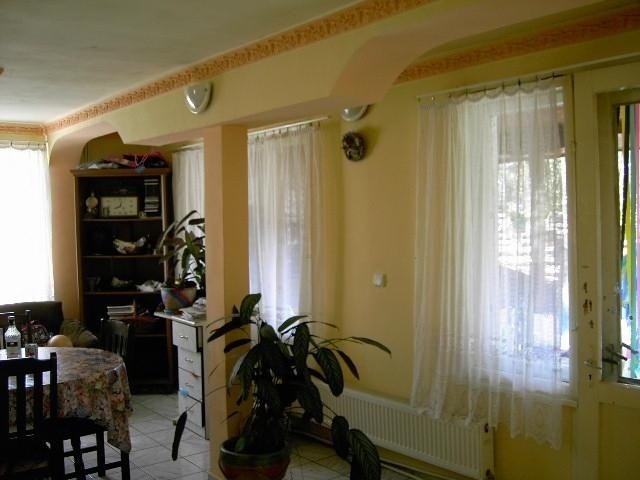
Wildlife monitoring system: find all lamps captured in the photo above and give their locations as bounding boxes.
[340,103,366,122]
[182,82,211,114]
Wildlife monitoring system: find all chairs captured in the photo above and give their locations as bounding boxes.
[62,318,133,480]
[0,309,34,349]
[0,351,64,480]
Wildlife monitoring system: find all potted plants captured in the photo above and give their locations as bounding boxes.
[151,207,207,312]
[170,291,391,480]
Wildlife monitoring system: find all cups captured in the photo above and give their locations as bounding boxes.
[25,344,38,358]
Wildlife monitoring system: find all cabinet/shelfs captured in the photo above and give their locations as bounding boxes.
[71,167,176,394]
[154,311,205,440]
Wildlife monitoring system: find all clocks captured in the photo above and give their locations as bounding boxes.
[99,194,140,219]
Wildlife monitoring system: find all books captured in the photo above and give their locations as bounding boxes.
[179,306,206,321]
[107,300,136,320]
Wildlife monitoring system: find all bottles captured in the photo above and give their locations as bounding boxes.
[4,316,21,359]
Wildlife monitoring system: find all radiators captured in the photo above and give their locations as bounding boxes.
[293,374,495,480]
